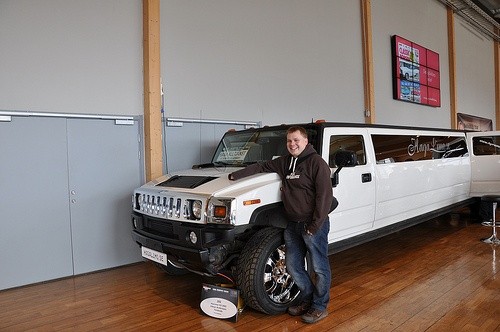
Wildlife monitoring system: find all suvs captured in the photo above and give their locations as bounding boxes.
[130,117,500,316]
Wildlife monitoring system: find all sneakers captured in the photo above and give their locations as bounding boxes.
[301,307,329,324]
[287,301,312,316]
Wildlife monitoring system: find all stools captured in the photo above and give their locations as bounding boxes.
[481,195,500,245]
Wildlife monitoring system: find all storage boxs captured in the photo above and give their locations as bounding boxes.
[198,272,247,324]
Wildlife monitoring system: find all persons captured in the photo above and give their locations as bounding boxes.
[229,125,333,323]
